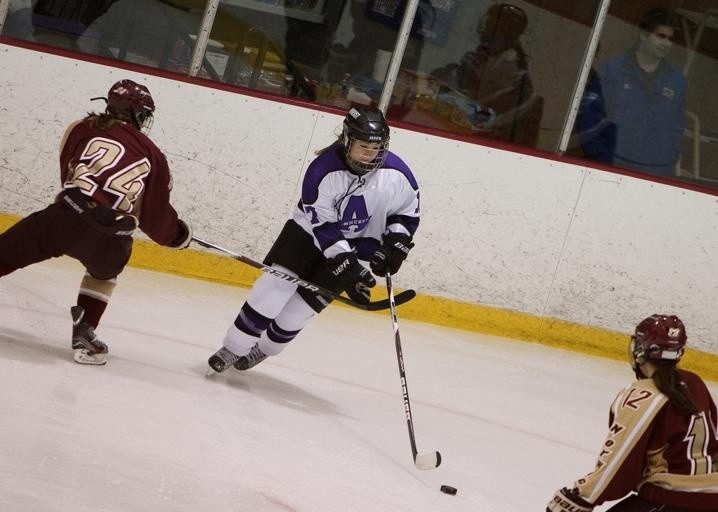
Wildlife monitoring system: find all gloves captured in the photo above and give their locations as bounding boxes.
[372,231,416,280]
[170,218,193,254]
[326,249,378,308]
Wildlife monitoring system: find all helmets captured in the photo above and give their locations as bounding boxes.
[341,103,392,158]
[632,312,688,365]
[108,77,155,121]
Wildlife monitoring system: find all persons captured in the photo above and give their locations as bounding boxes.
[283,1,347,102]
[425,2,536,143]
[29,0,114,46]
[348,1,436,124]
[0,78,194,354]
[545,313,718,511]
[208,104,421,373]
[576,8,692,179]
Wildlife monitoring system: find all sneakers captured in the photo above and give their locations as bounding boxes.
[207,346,240,373]
[232,342,271,370]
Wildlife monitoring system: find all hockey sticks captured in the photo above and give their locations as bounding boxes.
[381,232,441,469]
[191,236,416,311]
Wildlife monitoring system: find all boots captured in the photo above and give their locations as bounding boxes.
[68,301,109,356]
